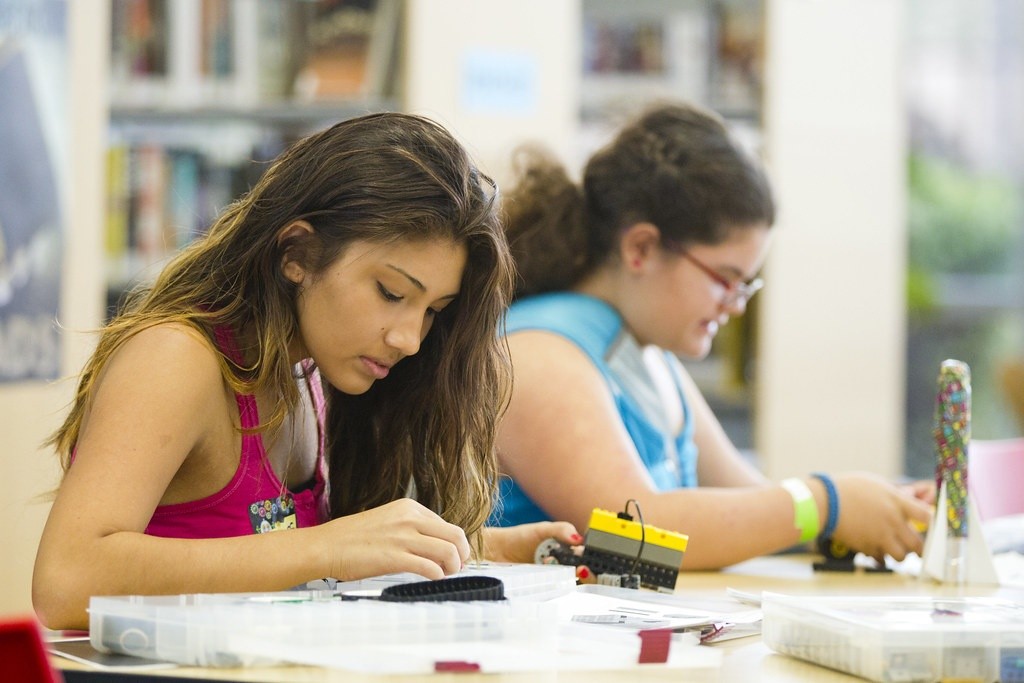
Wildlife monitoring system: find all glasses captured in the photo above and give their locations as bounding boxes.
[659,238,763,306]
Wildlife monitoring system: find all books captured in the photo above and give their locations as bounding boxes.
[103,0,404,330]
[580,1,768,454]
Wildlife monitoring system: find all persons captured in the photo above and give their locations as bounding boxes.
[471,96,940,574]
[28,110,587,641]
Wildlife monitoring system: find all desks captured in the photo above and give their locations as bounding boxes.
[43,550,1023,683]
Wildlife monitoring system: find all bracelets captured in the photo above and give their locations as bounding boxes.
[808,472,840,544]
[777,476,820,544]
